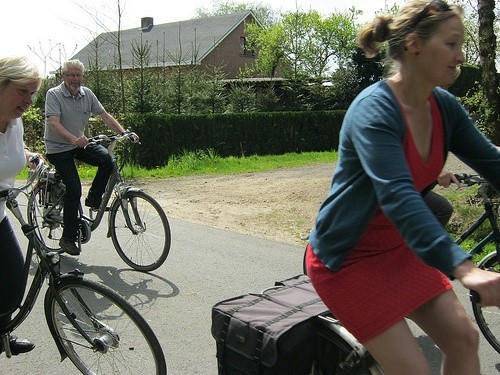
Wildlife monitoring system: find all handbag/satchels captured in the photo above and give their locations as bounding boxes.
[211,274,342,375]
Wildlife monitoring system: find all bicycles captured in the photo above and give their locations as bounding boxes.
[1,154,167,375]
[431,179,500,353]
[26,129,172,272]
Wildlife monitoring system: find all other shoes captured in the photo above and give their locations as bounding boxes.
[58,237,80,255]
[1,335,36,353]
[84,197,112,212]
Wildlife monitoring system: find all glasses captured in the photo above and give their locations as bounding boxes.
[412,0,448,28]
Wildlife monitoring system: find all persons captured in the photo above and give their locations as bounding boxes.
[305,0,500,375]
[0,55,42,354]
[45,59,139,255]
